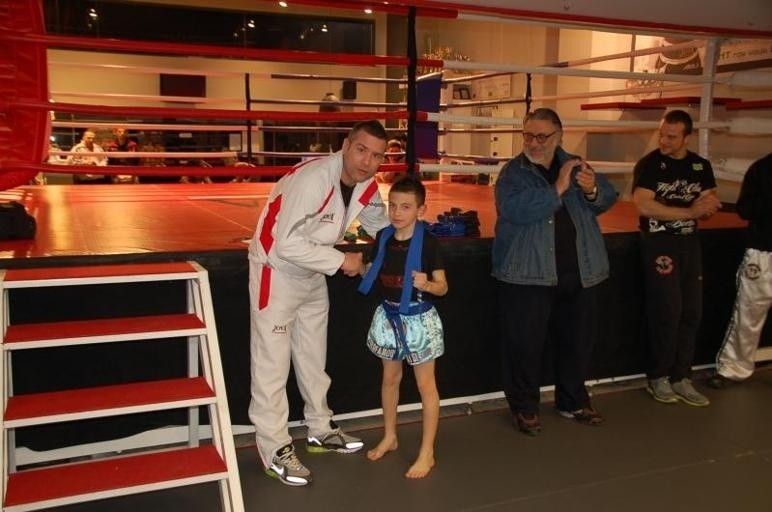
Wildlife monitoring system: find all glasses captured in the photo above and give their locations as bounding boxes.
[522,130,557,145]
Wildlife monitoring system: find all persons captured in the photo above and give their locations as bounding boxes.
[379,132,406,177]
[180,148,253,184]
[344,178,448,479]
[65,127,164,184]
[248,120,391,486]
[490,108,617,436]
[710,151,772,391]
[631,109,722,407]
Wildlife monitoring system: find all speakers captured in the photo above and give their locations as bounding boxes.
[344,79,356,100]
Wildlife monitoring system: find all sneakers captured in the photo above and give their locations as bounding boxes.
[430,207,479,237]
[648,372,729,406]
[553,399,605,425]
[263,441,313,487]
[306,420,365,453]
[516,409,542,434]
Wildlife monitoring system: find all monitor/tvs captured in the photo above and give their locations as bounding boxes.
[159,73,207,98]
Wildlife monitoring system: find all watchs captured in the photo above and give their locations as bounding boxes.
[585,185,597,200]
